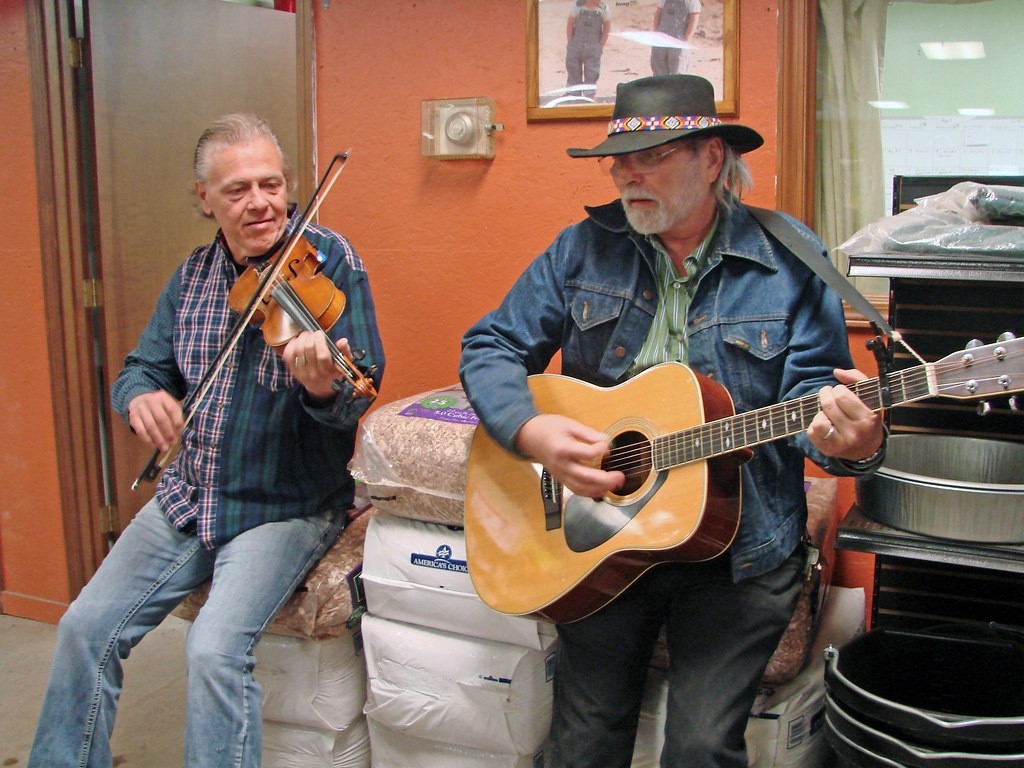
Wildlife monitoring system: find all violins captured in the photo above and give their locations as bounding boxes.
[228,232,379,400]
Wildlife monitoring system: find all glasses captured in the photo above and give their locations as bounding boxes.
[598,142,689,175]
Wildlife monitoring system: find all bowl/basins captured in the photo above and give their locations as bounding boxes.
[854,433,1024,544]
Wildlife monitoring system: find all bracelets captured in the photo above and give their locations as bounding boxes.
[855,447,882,465]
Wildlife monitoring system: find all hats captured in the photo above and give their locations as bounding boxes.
[566,73,764,159]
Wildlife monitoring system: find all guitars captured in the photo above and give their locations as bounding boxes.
[461,333,1023,621]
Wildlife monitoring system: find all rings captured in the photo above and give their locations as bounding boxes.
[821,425,835,440]
[294,356,306,366]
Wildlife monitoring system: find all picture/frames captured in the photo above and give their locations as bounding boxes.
[525,0,741,125]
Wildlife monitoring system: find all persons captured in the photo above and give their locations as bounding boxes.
[563,1,611,101]
[649,0,702,77]
[25,111,385,768]
[458,72,893,768]
